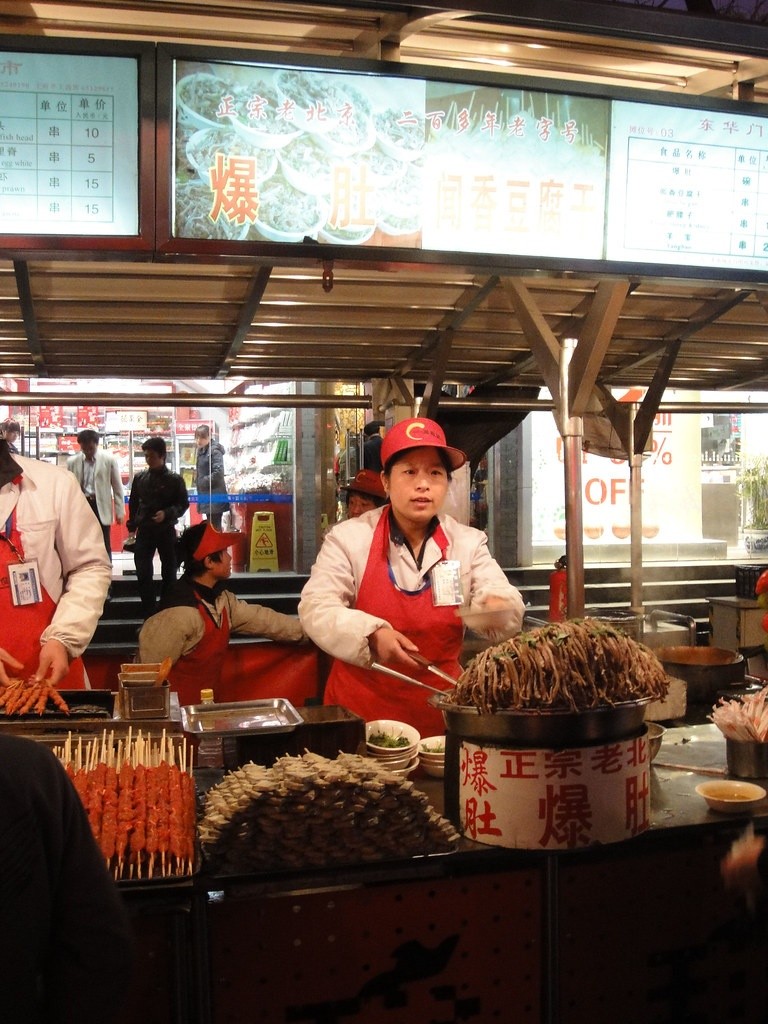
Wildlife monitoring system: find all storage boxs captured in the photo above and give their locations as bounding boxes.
[221,705,371,774]
[116,673,171,719]
[735,564,768,600]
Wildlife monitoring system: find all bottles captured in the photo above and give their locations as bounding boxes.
[200,689,215,705]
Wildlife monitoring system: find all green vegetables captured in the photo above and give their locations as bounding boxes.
[490,618,627,663]
[367,728,445,753]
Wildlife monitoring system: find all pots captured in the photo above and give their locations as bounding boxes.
[430,690,659,747]
[654,645,746,704]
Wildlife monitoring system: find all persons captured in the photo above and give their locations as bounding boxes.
[333,421,385,478]
[16,573,28,582]
[127,437,189,636]
[323,469,389,534]
[295,418,527,741]
[194,425,231,528]
[0,437,113,690]
[66,430,127,563]
[0,735,133,1023]
[137,522,324,707]
[0,418,21,455]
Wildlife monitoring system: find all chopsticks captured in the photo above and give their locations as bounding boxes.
[707,690,767,741]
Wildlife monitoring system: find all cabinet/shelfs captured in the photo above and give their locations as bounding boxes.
[222,380,294,495]
[705,596,768,654]
[15,408,216,494]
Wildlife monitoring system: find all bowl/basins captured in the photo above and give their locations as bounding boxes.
[695,781,767,812]
[366,719,448,781]
[177,69,423,245]
[648,721,668,763]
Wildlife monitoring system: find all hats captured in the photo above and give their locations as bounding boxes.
[340,470,386,501]
[380,418,466,471]
[185,520,245,566]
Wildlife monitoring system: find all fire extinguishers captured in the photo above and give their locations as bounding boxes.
[549,553,569,625]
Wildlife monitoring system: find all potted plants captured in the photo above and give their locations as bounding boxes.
[733,455,768,555]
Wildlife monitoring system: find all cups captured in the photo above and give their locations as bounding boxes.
[725,736,768,779]
